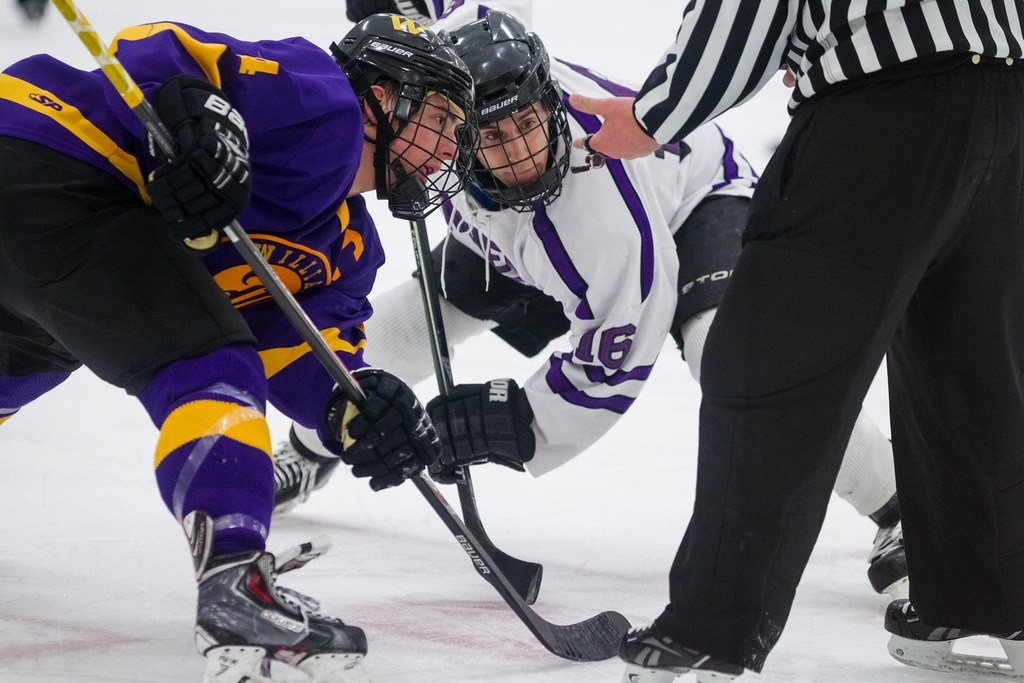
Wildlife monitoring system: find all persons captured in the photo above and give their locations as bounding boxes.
[570,0,1023,675]
[0,12,483,534]
[270,0,924,594]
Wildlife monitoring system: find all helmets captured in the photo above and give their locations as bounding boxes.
[326,12,481,222]
[441,10,573,213]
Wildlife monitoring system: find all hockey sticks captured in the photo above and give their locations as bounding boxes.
[408,214,546,608]
[50,0,634,664]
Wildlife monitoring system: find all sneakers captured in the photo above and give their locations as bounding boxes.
[182,510,368,683]
[269,419,341,515]
[617,621,745,683]
[868,476,910,606]
[884,594,1024,683]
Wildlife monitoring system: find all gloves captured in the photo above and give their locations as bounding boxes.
[138,74,252,242]
[426,378,536,486]
[328,368,443,491]
